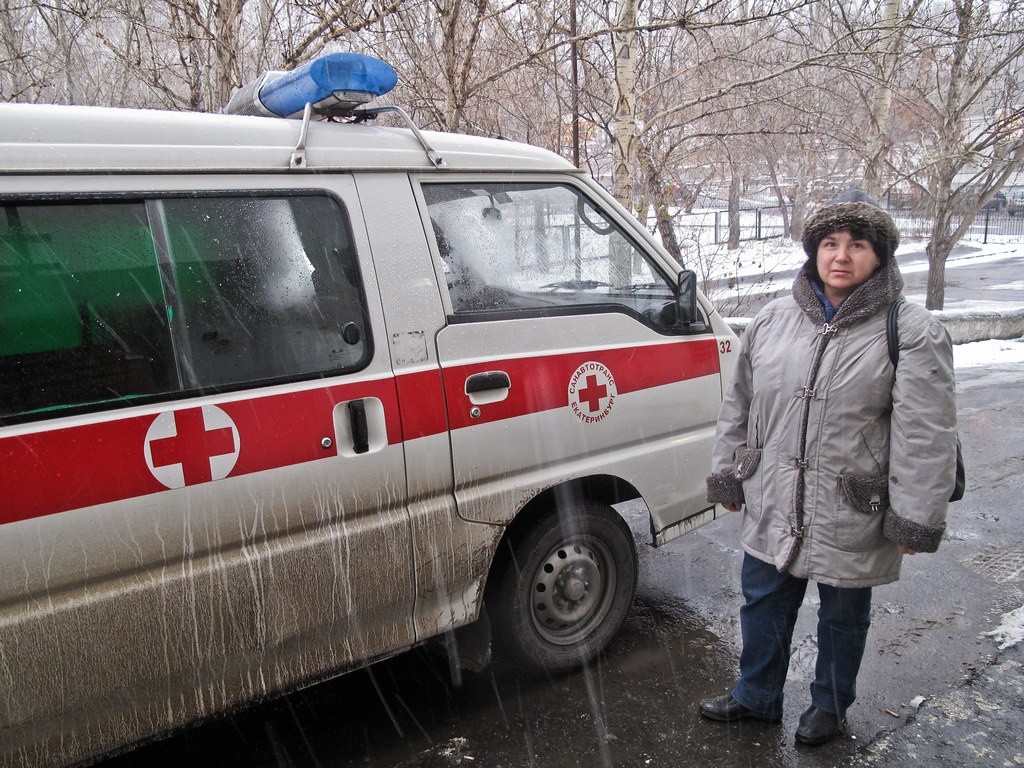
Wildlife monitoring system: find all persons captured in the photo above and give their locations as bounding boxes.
[698,188,959,745]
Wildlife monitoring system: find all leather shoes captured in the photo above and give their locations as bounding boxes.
[700,691,783,724]
[795,705,847,744]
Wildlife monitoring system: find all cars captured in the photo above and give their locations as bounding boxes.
[1006,190,1024,215]
[889,192,919,208]
[674,182,694,200]
[787,179,838,203]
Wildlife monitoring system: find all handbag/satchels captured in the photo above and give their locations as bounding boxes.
[886,299,965,503]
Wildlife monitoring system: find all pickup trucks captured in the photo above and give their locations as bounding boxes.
[948,184,1008,213]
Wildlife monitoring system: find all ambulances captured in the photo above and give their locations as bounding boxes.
[1,53,742,768]
